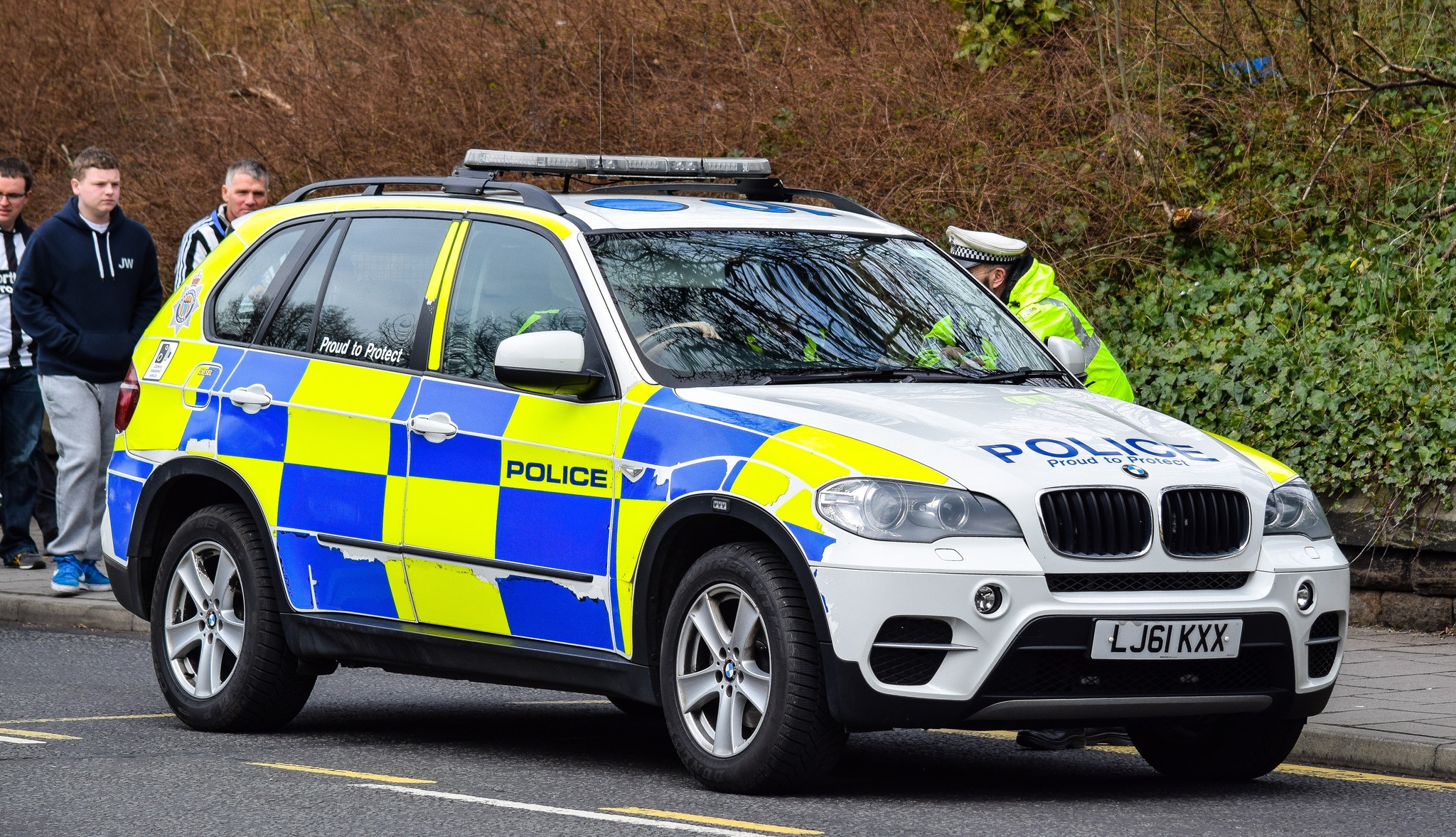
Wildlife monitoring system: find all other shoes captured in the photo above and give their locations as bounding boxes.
[1083,727,1134,746]
[1016,729,1086,751]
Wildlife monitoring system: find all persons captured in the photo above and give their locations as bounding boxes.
[0,154,62,572]
[173,161,273,295]
[911,226,1138,407]
[730,260,909,371]
[502,263,726,395]
[15,146,161,595]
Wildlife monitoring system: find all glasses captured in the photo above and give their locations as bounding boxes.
[0,191,26,201]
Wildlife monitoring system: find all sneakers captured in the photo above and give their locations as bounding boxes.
[49,555,84,593]
[79,560,113,592]
[3,547,47,570]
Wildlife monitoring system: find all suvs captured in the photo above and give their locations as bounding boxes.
[98,146,1352,795]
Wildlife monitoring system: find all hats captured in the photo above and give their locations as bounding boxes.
[946,225,1028,269]
